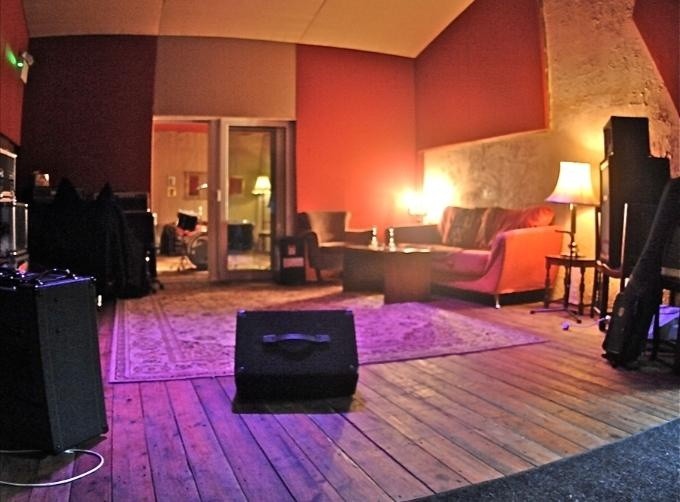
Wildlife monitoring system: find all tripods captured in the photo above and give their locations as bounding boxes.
[170,230,195,272]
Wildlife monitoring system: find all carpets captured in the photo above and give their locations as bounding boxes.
[398,418,680,501]
[109,278,550,384]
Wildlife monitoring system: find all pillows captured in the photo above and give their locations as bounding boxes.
[441,202,553,250]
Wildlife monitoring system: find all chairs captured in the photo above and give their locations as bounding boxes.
[596,200,662,340]
[295,210,377,282]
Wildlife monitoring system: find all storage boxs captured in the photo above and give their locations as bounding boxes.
[0,148,31,262]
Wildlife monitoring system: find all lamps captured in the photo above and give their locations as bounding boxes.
[17,51,34,84]
[544,160,597,257]
[251,177,272,232]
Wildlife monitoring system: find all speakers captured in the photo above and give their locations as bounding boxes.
[233,308,359,397]
[0,271,109,453]
[602,115,651,158]
[598,156,670,271]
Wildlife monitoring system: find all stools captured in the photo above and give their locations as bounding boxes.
[650,263,680,370]
[543,254,597,317]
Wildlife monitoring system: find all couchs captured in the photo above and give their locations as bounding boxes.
[385,207,565,309]
